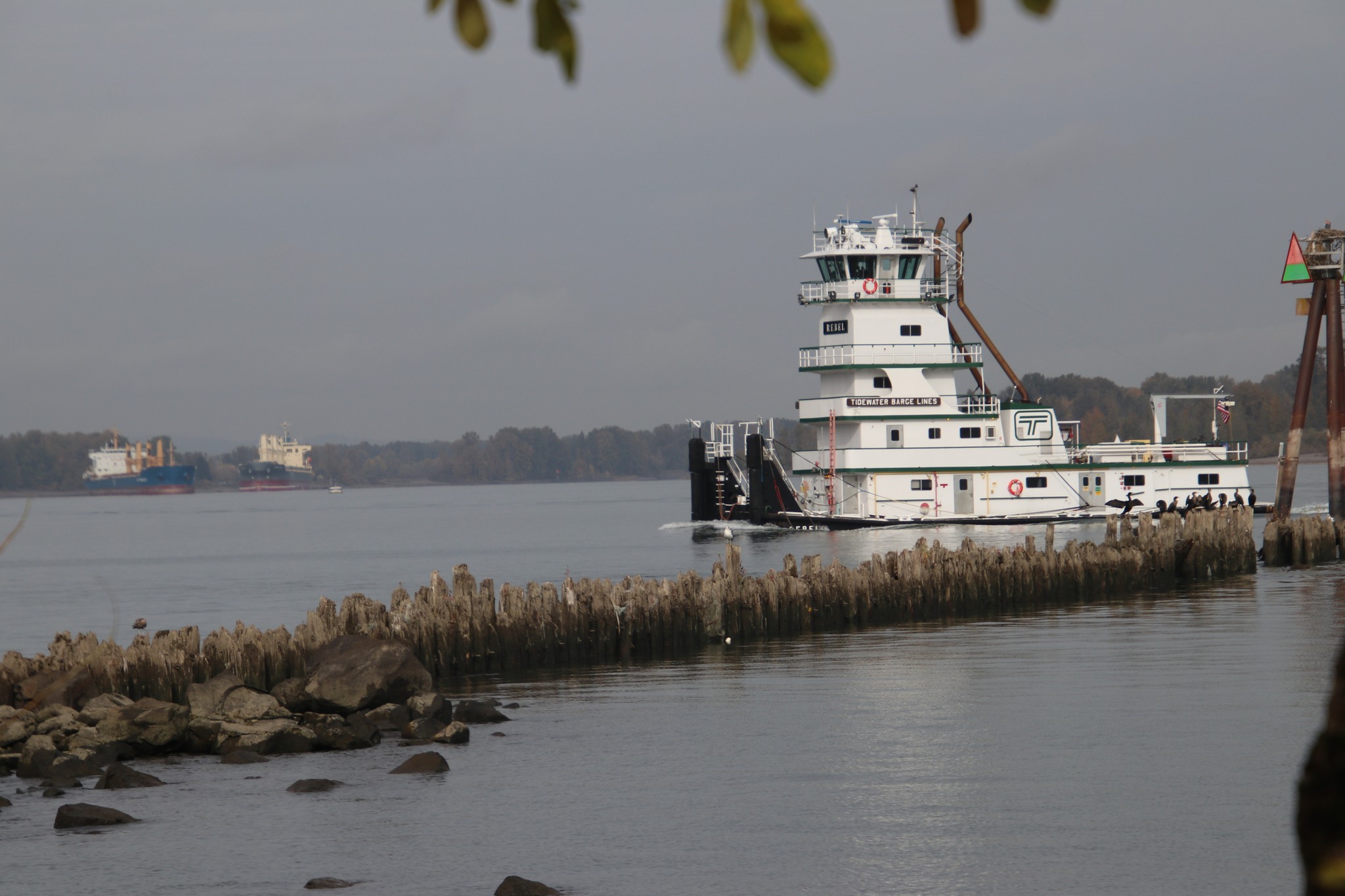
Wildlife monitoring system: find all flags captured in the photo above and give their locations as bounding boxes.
[1217,390,1230,424]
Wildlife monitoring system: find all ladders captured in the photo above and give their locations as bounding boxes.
[829,409,835,513]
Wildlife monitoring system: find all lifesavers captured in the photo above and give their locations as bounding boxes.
[863,279,877,295]
[1008,479,1023,495]
[828,492,830,505]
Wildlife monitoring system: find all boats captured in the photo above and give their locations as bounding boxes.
[328,484,342,493]
[686,184,1276,528]
[80,428,198,495]
[238,420,330,493]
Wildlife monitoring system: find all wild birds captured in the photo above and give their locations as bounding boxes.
[131,618,146,636]
[1234,488,1246,510]
[1248,488,1257,509]
[1219,493,1227,506]
[724,526,734,544]
[1156,488,1212,513]
[1105,492,1144,515]
